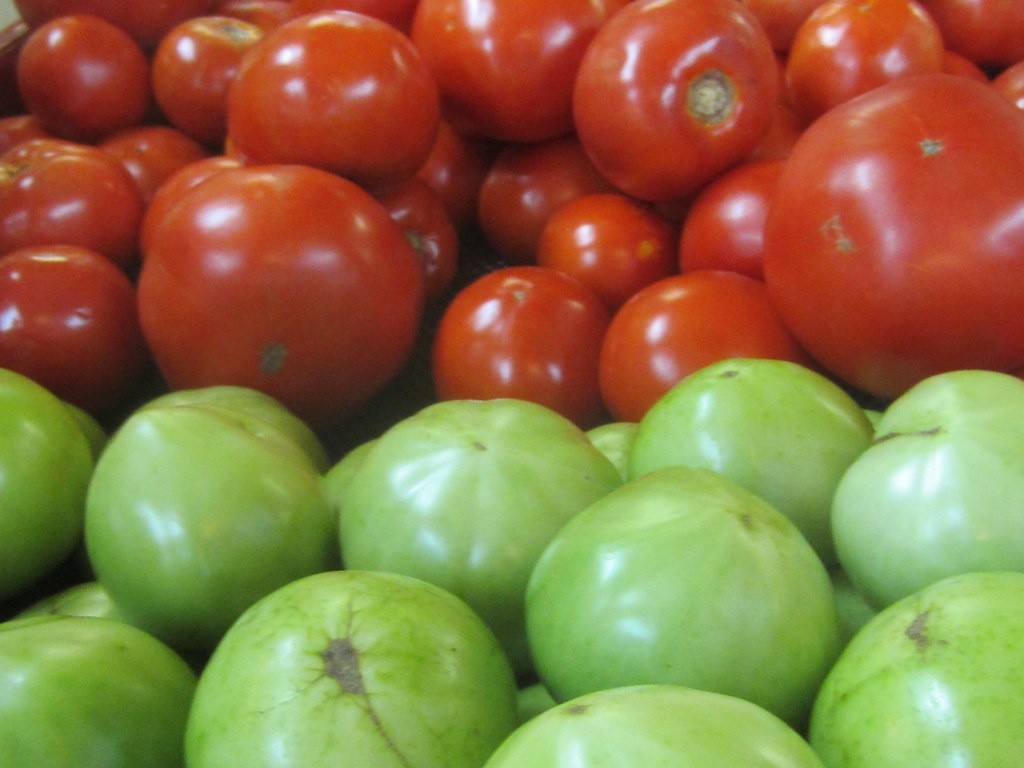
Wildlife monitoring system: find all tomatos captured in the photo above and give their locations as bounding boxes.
[0,358,1024,768]
[0,0,1024,449]
